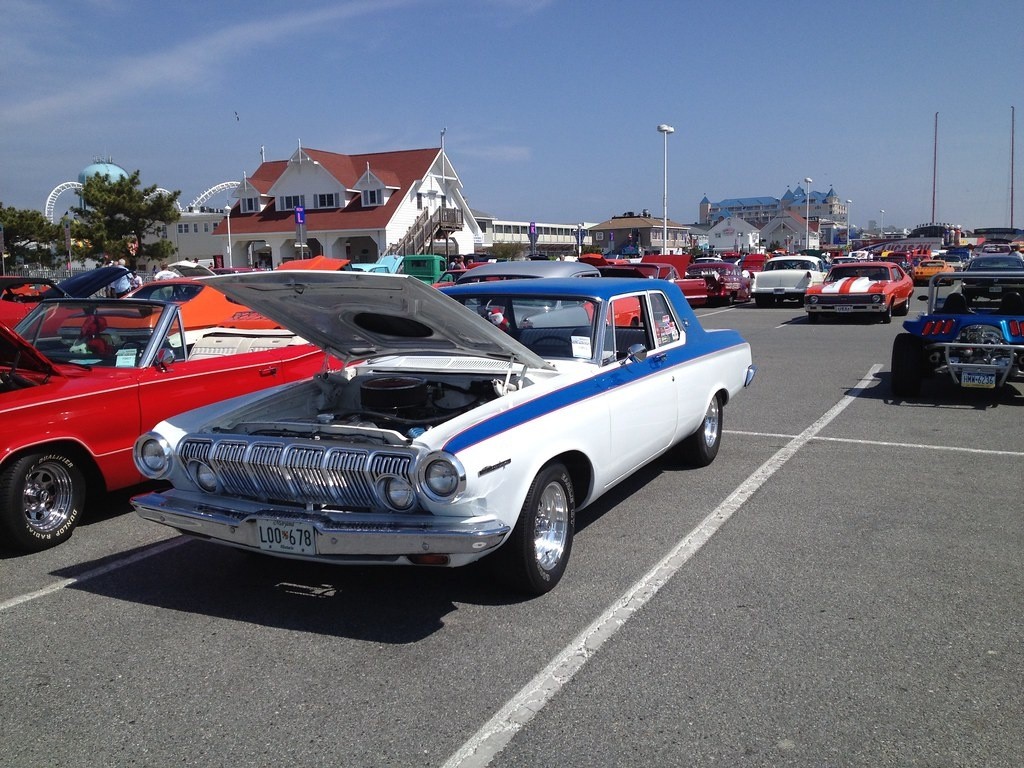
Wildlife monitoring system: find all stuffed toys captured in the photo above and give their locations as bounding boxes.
[489,307,510,333]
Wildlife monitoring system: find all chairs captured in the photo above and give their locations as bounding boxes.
[997,292,1022,317]
[935,294,976,316]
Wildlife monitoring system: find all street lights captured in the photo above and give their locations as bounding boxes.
[804,177,812,251]
[879,209,885,238]
[845,200,853,248]
[737,232,742,253]
[224,202,236,266]
[658,123,675,256]
[576,223,583,259]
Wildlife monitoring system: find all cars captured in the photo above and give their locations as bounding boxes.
[890,268,1024,403]
[0,296,368,555]
[961,254,1024,299]
[913,259,955,286]
[754,255,829,306]
[940,255,963,272]
[0,251,782,359]
[127,269,755,593]
[822,242,1024,283]
[803,261,914,324]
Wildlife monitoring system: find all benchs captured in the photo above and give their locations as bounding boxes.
[186,330,291,361]
[521,328,648,353]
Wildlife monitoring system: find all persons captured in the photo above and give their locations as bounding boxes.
[1009,244,1024,262]
[94,257,283,300]
[559,254,565,261]
[450,255,474,282]
